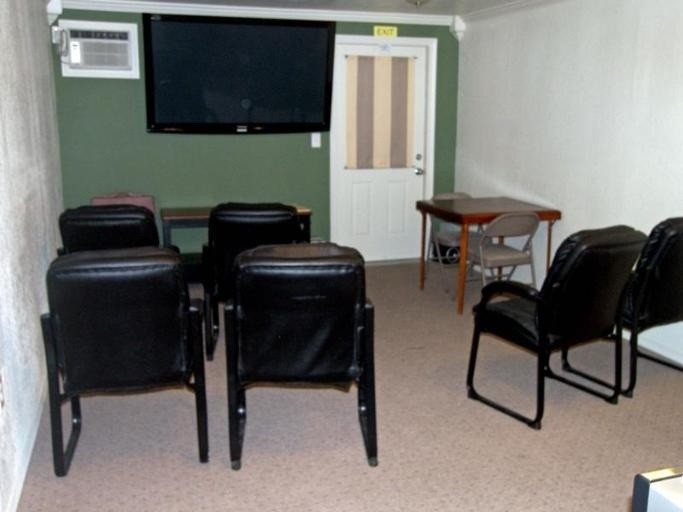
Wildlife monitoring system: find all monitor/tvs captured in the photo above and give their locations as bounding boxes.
[141,11,338,135]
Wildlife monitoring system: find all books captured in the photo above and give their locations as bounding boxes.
[628,465,682,512]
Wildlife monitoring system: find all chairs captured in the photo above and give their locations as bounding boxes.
[424,191,540,305]
